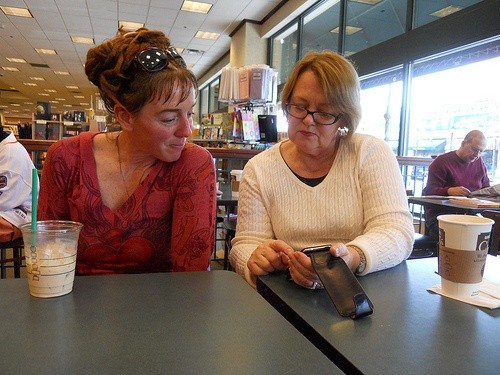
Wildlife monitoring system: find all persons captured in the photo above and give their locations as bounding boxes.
[227,50,415,290]
[421,129,490,241]
[0,116,41,243]
[37,29,217,277]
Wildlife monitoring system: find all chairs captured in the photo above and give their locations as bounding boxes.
[483,210,500,257]
[0,237,27,278]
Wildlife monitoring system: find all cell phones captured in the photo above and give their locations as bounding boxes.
[288,245,331,271]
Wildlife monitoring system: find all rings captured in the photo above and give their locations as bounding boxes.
[311,282,319,290]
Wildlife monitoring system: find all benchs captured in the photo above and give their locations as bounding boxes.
[408,233,437,260]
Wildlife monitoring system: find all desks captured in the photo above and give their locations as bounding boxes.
[0,270,347,375]
[408,195,500,215]
[256,254,500,375]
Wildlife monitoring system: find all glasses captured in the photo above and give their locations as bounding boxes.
[286,102,342,125]
[124,47,187,77]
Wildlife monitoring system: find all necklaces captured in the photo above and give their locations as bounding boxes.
[115,130,145,198]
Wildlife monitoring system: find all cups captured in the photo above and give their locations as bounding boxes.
[229,169,242,198]
[18,220,83,299]
[437,214,495,297]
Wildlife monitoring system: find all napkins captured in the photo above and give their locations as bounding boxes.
[427,256,500,309]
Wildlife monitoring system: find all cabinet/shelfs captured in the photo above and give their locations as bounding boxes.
[32,112,81,174]
